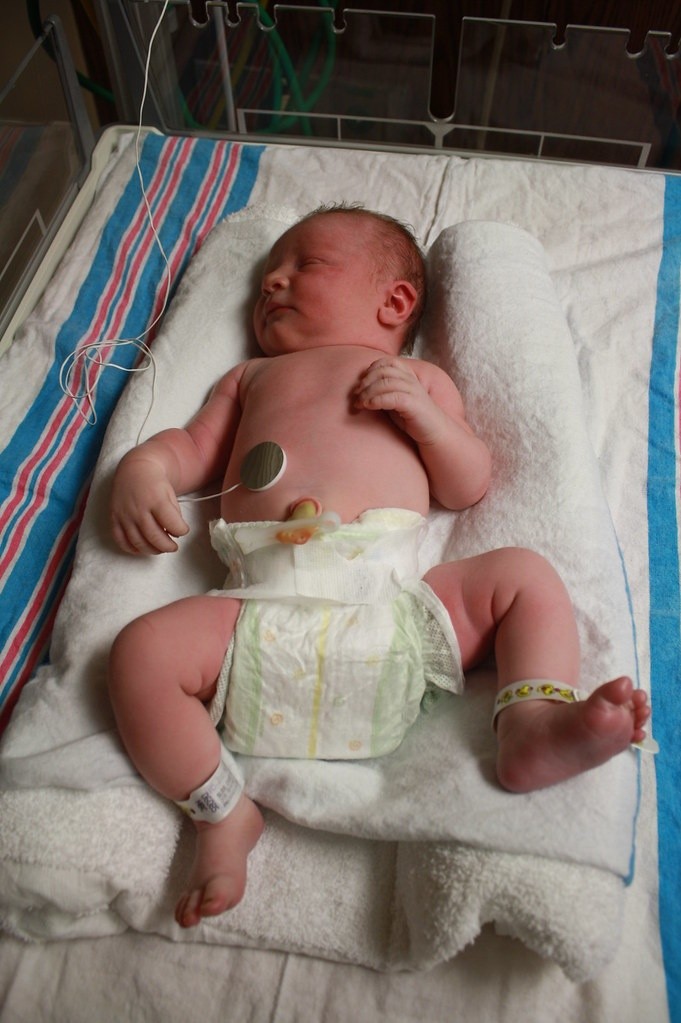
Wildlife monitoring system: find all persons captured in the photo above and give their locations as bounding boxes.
[103,200,653,928]
